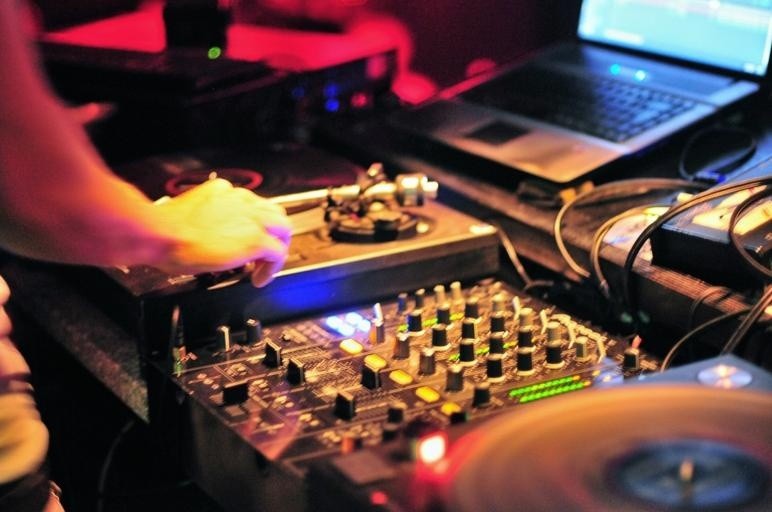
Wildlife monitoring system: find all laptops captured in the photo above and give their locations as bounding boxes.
[386,0,772,184]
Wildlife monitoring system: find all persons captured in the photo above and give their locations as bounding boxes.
[0,1,291,511]
